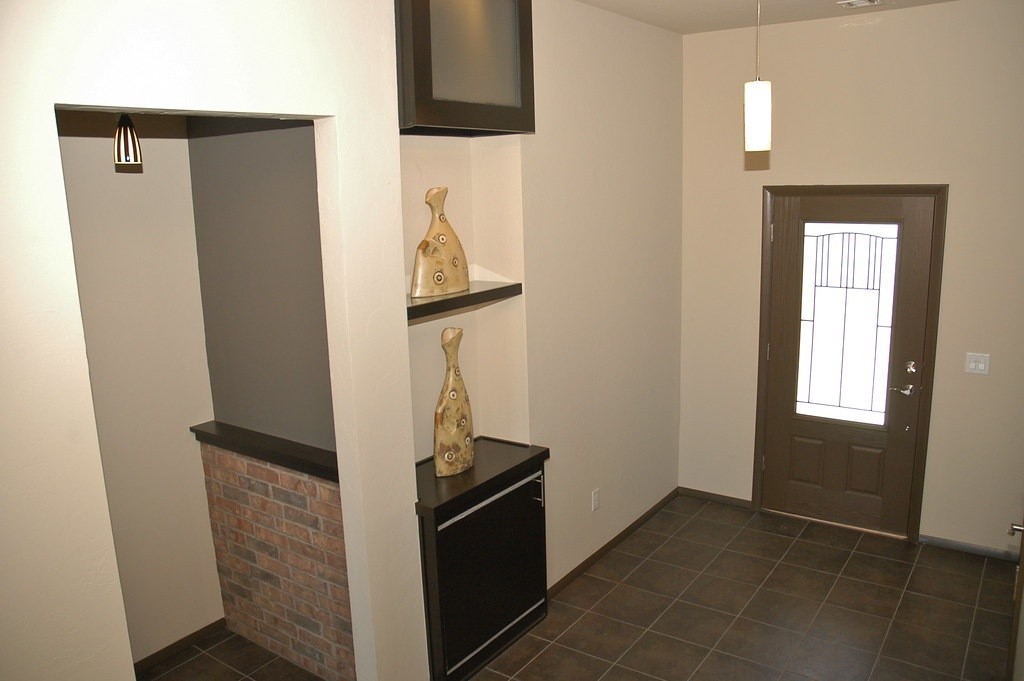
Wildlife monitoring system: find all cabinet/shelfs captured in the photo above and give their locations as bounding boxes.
[394,0,535,137]
[413,436,551,681]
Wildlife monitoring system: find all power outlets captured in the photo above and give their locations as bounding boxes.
[591,488,600,512]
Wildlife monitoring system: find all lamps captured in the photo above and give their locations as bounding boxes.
[744,0,771,151]
[112,113,144,174]
